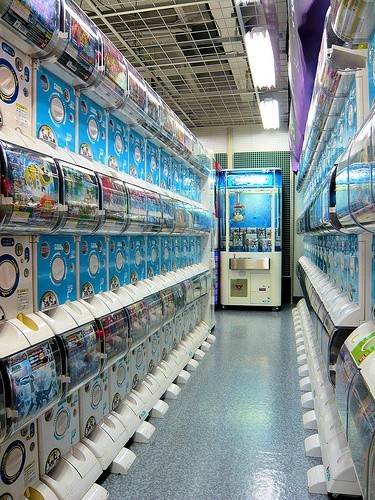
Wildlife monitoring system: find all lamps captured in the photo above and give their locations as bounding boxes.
[258,97,280,130]
[244,27,277,89]
